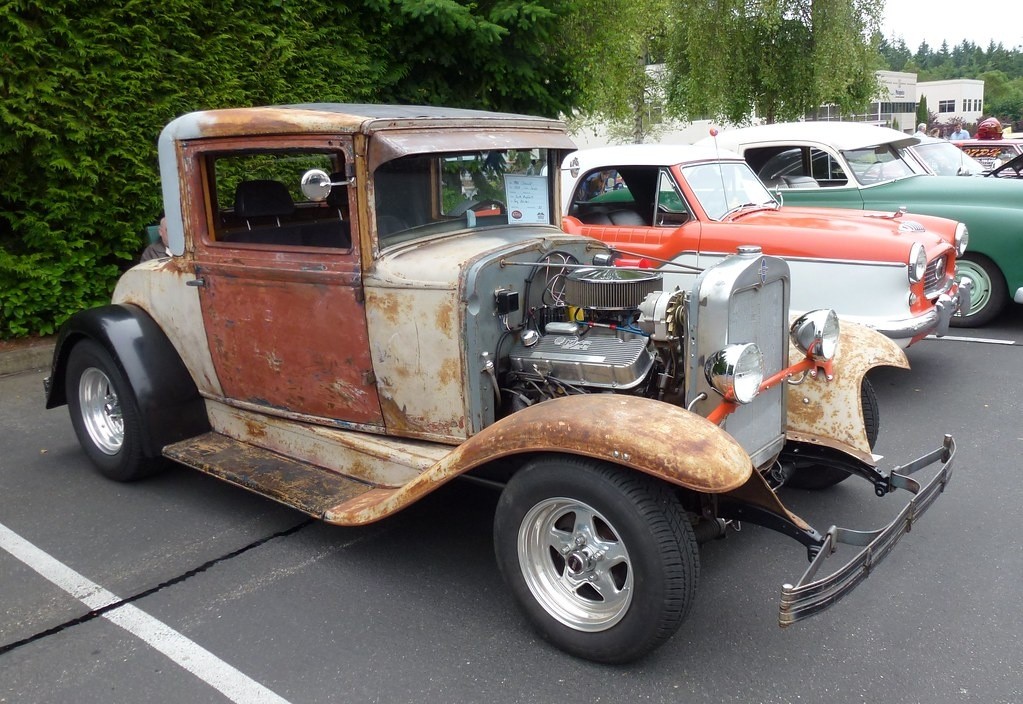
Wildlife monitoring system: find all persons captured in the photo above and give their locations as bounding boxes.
[950,123,970,140]
[929,128,939,138]
[587,170,611,201]
[913,123,927,137]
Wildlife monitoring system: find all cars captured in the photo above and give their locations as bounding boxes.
[933,126,1023,181]
[46,103,957,665]
[568,118,1023,329]
[450,143,970,357]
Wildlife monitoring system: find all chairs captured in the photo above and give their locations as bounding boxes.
[224,173,407,250]
[762,175,821,189]
[579,210,649,226]
